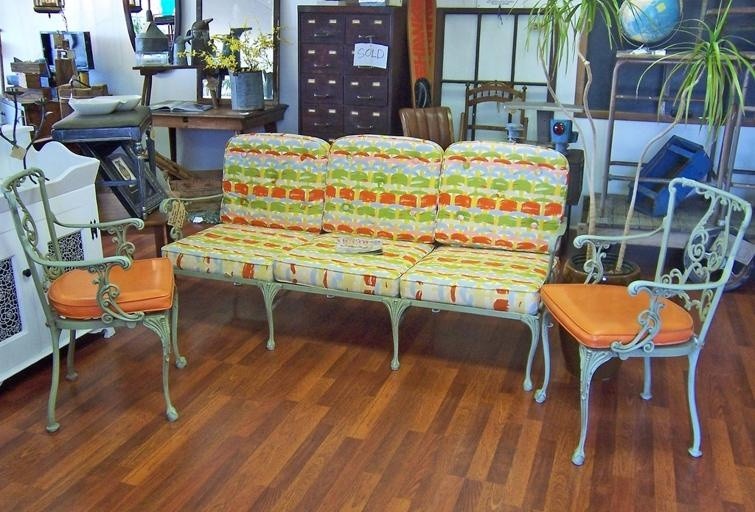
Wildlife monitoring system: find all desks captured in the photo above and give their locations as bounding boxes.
[152,104,289,164]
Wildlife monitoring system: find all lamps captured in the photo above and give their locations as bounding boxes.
[134,1,169,67]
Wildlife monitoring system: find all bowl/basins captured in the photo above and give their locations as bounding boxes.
[68,95,142,114]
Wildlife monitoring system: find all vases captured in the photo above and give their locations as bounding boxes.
[231,71,264,111]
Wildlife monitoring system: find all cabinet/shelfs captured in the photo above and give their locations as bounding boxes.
[298,6,412,146]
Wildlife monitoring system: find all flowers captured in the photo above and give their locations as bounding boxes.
[178,18,282,74]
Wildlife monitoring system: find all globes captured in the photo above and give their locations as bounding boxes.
[617,0,685,56]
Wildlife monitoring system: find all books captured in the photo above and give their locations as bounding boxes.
[148,99,214,114]
[316,1,356,7]
[358,1,387,7]
[337,236,383,257]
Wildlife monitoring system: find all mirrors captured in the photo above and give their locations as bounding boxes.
[195,0,281,107]
[122,0,182,55]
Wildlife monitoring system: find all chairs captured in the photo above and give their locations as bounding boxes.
[397,107,455,152]
